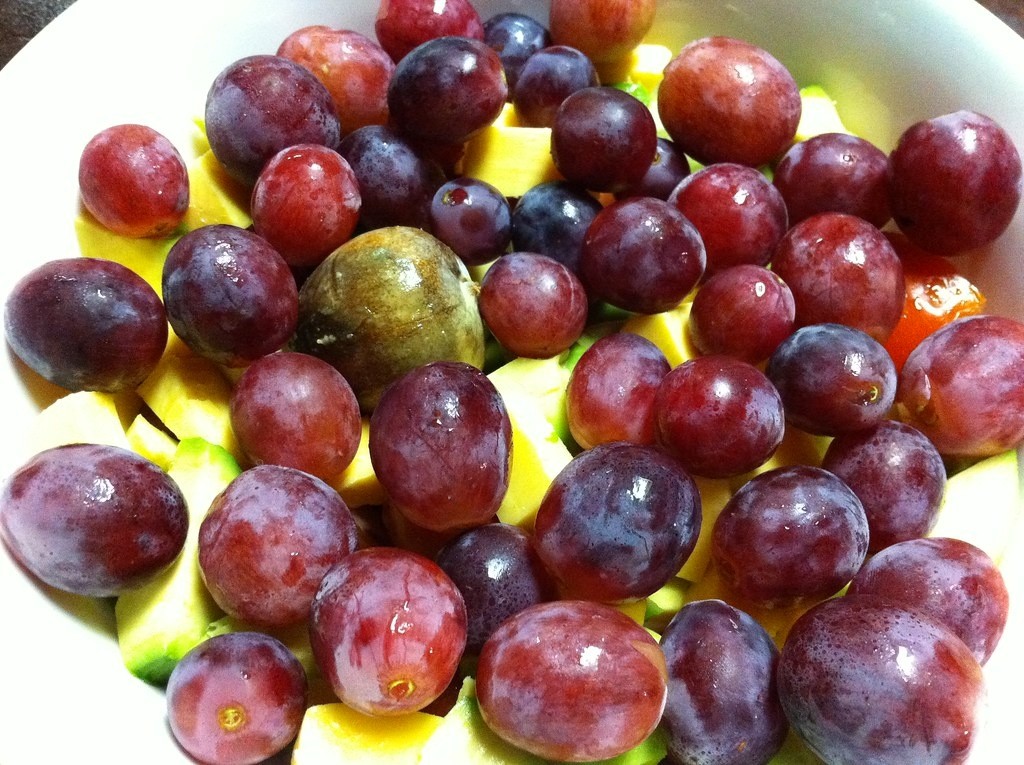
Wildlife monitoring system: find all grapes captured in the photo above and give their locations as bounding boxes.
[0,0,1024,765]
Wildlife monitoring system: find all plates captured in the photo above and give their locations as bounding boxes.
[0,0,1024,765]
